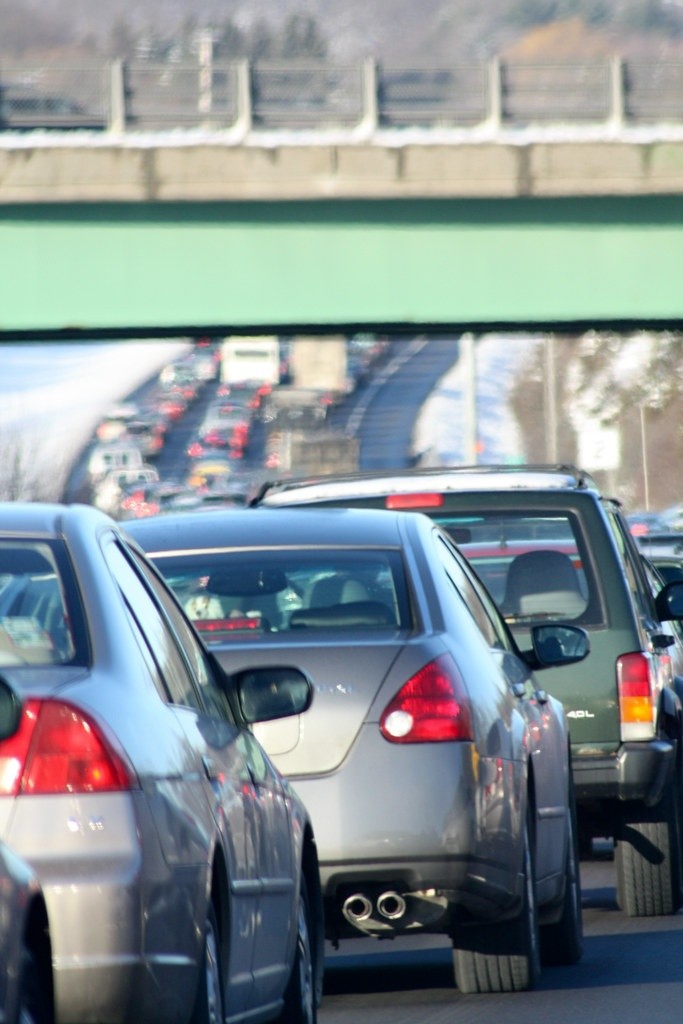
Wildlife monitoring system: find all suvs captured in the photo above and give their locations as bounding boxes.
[220,461,683,917]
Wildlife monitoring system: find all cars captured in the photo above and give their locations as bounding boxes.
[0,522,325,1024]
[2,502,587,999]
[82,337,683,682]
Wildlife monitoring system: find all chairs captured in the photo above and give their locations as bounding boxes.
[497,550,588,622]
[303,576,384,611]
